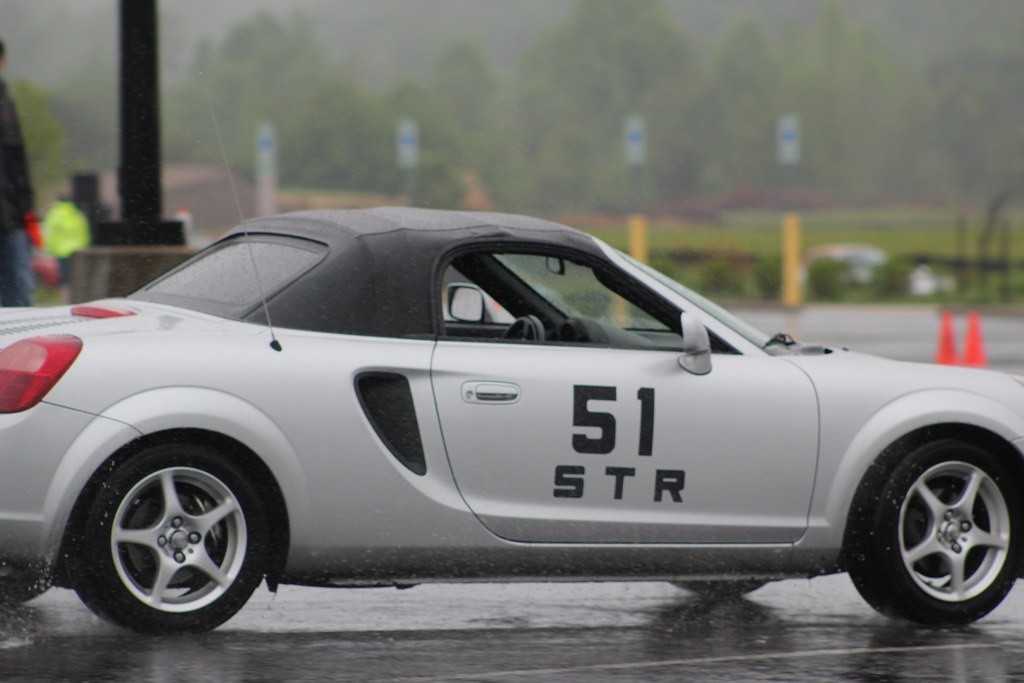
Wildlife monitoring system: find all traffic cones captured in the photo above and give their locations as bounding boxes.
[932,308,958,366]
[962,306,990,367]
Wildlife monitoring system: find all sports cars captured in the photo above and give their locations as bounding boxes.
[0,206,1024,639]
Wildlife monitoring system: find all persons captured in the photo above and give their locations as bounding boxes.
[39,186,92,302]
[0,39,42,307]
[178,206,194,234]
[837,252,959,302]
